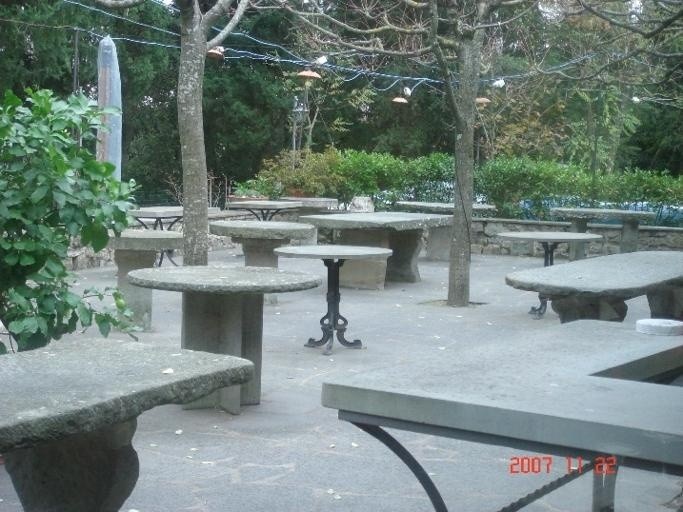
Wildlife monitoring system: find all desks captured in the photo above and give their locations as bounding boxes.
[505,251,682,323]
[127,265,323,416]
[322,318,682,511]
[549,208,656,262]
[299,211,454,284]
[0,335,255,511]
[273,245,393,355]
[394,202,497,214]
[224,202,304,221]
[495,231,603,267]
[126,207,220,267]
[103,229,183,333]
[209,220,316,306]
